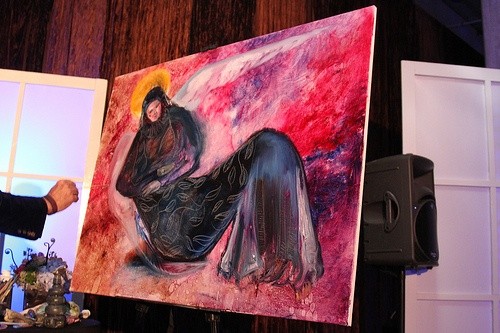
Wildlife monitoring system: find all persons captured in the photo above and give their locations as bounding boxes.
[0,179,79,241]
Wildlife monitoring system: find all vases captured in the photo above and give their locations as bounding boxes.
[23,288,51,310]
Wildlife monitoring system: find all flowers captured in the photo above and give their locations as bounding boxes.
[0,252,72,293]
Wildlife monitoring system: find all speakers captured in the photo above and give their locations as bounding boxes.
[364,154,440,266]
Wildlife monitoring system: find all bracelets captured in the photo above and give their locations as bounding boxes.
[44,195,57,214]
